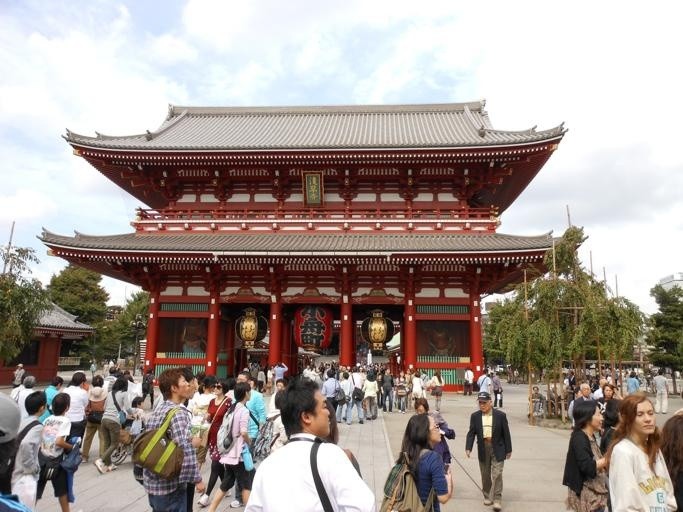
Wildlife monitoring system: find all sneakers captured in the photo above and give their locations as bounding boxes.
[484,498,493,505]
[197,493,209,507]
[93,458,106,474]
[229,500,245,508]
[337,410,405,426]
[107,464,117,472]
[492,499,501,510]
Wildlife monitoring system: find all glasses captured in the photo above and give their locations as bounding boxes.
[430,424,440,432]
[213,385,222,390]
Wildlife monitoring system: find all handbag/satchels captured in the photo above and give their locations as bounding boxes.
[0,420,43,495]
[431,386,442,396]
[87,411,103,424]
[132,407,184,480]
[118,410,126,425]
[200,430,209,447]
[345,395,351,403]
[352,387,365,401]
[397,390,408,396]
[39,462,62,481]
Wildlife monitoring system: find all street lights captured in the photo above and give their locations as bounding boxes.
[127,311,144,376]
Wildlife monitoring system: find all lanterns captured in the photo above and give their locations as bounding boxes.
[293,304,335,357]
[359,308,395,354]
[233,305,268,350]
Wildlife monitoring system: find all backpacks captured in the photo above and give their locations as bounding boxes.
[379,449,435,512]
[334,380,346,406]
[217,403,244,455]
[253,414,281,458]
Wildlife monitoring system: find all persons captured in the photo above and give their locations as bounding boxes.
[563,395,612,512]
[132,367,383,511]
[413,399,457,469]
[606,395,678,512]
[528,387,546,418]
[507,366,548,384]
[398,414,452,511]
[464,390,512,512]
[383,362,502,412]
[1,390,80,512]
[660,413,683,512]
[1,358,145,475]
[242,359,382,425]
[549,368,683,454]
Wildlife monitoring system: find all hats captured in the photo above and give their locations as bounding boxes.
[88,387,108,402]
[477,392,489,400]
[16,363,23,368]
